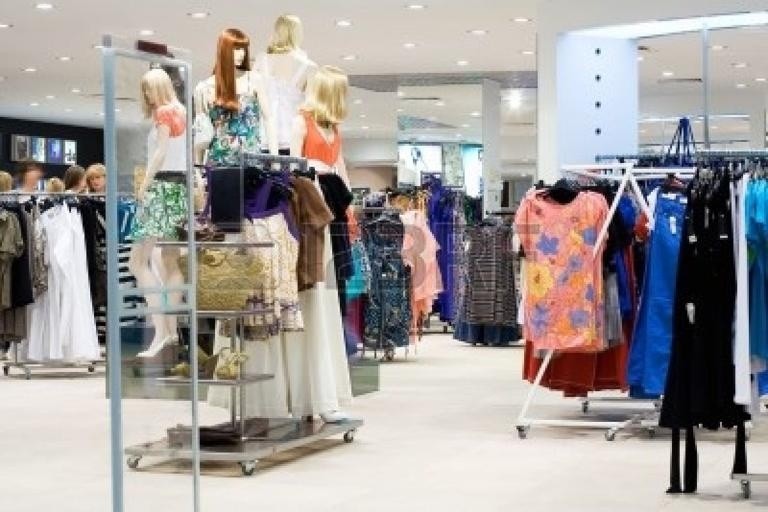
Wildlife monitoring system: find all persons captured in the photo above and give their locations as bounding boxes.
[411,147,429,184]
[145,54,206,228]
[124,68,191,360]
[251,12,317,154]
[0,151,106,214]
[289,62,357,290]
[188,27,285,230]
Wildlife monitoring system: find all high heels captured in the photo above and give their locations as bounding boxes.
[136,334,247,378]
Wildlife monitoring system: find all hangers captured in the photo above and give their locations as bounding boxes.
[352,184,427,213]
[0,190,130,203]
[239,149,309,173]
[536,149,768,202]
[478,209,499,225]
